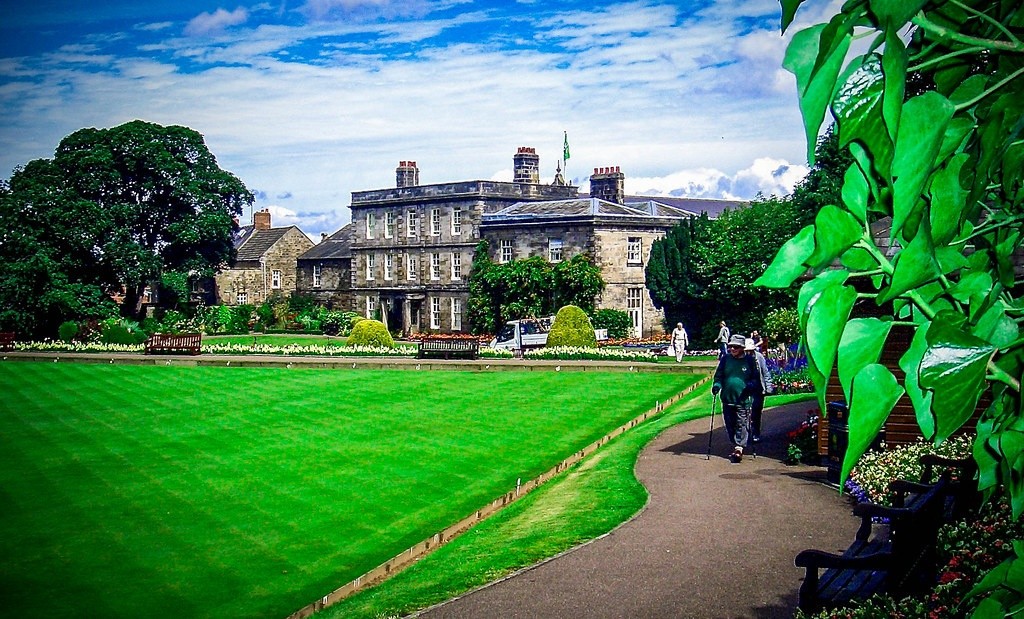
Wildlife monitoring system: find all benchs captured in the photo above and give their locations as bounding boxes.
[144,333,202,356]
[415,337,481,360]
[0,331,17,351]
[794,455,984,619]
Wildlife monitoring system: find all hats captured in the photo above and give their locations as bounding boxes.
[721,321,726,326]
[727,334,746,348]
[743,338,756,350]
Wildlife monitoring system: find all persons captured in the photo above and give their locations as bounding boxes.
[714,322,730,360]
[670,322,688,362]
[713,334,757,463]
[751,330,768,358]
[743,338,772,442]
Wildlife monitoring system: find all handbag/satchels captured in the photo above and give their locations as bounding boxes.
[667,345,675,356]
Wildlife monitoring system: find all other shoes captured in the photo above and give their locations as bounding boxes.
[752,434,759,442]
[731,445,743,463]
[717,358,720,360]
[677,360,681,363]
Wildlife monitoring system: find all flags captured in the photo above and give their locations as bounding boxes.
[563,138,570,160]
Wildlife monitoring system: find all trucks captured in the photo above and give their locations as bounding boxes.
[490,318,608,351]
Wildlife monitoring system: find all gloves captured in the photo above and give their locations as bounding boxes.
[712,386,720,395]
[739,387,751,401]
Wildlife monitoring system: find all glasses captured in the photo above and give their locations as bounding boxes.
[730,345,741,349]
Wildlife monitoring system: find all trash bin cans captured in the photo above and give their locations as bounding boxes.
[826,400,887,485]
